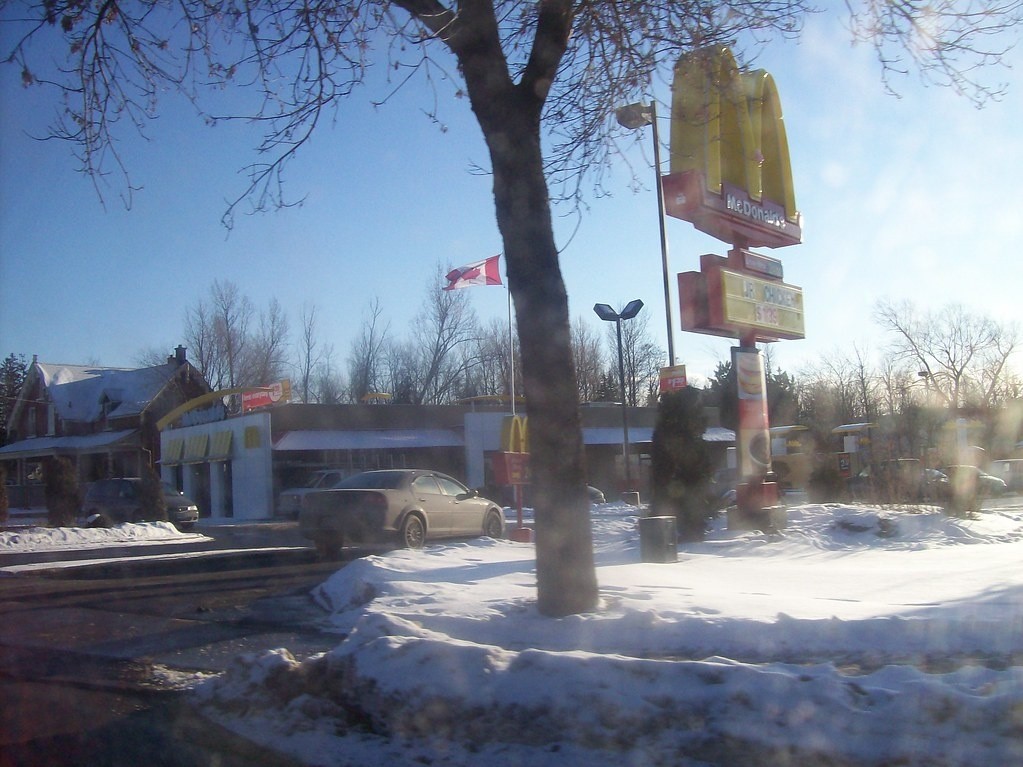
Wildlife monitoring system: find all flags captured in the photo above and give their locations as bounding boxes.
[442,253,503,292]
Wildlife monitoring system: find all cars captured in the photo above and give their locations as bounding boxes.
[277,470,346,515]
[80,476,200,531]
[923,469,952,502]
[943,465,1008,498]
[298,469,507,564]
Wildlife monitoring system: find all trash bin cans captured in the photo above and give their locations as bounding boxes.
[638,514,679,563]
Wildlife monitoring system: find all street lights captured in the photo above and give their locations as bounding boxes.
[917,370,931,447]
[593,299,645,505]
[613,98,673,366]
